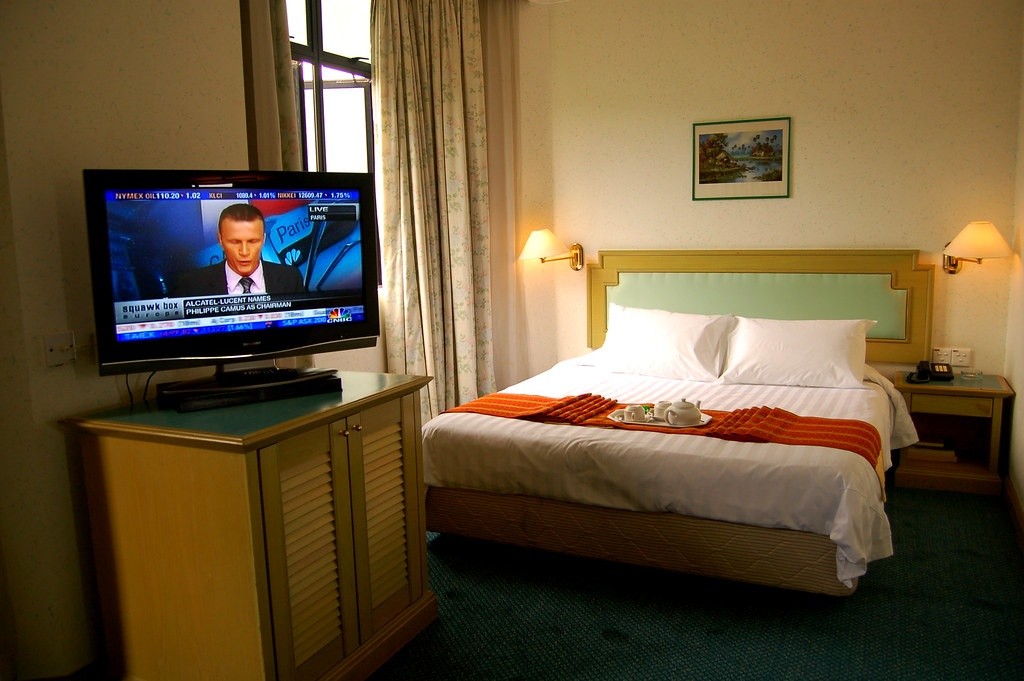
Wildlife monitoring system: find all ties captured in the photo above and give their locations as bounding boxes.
[239,278,254,293]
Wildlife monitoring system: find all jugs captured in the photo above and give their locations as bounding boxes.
[664,398,701,426]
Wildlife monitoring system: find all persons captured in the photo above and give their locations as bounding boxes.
[173,204,303,297]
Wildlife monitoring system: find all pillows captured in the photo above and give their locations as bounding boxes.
[595,300,738,383]
[724,312,879,389]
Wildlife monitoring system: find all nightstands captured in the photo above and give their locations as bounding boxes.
[889,368,1014,497]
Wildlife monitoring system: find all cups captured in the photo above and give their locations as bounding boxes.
[654,401,672,419]
[624,404,646,422]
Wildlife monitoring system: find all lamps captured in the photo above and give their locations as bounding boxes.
[943,220,1013,273]
[517,229,583,271]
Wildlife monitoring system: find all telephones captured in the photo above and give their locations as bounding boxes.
[906,360,955,384]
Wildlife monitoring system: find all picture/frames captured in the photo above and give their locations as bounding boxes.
[692,116,790,201]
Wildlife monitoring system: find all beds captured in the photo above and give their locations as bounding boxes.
[424,248,944,598]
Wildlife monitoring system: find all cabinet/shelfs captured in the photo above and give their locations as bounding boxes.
[58,369,430,681]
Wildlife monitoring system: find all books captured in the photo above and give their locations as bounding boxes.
[907,434,958,463]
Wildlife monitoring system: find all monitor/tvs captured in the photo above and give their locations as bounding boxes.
[81,167,380,410]
